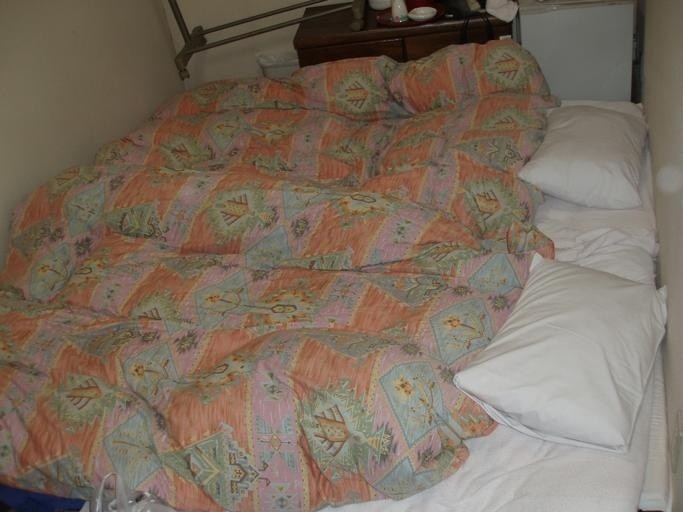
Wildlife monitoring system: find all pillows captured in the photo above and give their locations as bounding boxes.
[516,101,651,212]
[450,249,669,458]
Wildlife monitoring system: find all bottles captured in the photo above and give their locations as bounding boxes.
[392,1,409,23]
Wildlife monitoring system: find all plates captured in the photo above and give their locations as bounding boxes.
[409,7,437,21]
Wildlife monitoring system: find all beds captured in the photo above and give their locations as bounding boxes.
[0,83,682,511]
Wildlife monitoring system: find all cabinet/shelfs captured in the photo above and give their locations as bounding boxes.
[293,2,515,71]
